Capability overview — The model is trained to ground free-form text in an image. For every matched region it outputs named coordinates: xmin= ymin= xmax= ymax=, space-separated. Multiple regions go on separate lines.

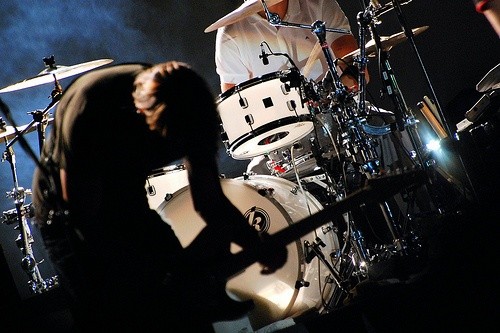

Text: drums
xmin=266 ymin=110 xmax=346 ymax=180
xmin=145 ymin=163 xmax=189 ymax=209
xmin=156 ymin=172 xmax=341 ymax=331
xmin=213 ymin=69 xmax=318 ymax=161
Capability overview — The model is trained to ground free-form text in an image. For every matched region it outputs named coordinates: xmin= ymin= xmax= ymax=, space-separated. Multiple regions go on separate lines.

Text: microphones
xmin=260 ymin=43 xmax=269 ymax=65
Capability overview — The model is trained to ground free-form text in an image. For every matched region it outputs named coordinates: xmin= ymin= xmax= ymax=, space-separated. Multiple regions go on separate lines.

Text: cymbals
xmin=204 ymin=0 xmax=284 ymax=33
xmin=0 ymin=58 xmax=114 ymax=94
xmin=337 ymin=25 xmax=428 ymax=66
xmin=0 ymin=117 xmax=54 ymax=142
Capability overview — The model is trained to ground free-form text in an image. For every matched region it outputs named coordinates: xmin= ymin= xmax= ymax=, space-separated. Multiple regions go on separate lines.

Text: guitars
xmin=62 ymin=165 xmax=427 ymax=331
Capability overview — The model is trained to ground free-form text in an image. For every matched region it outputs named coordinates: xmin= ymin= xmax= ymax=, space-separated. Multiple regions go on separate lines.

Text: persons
xmin=32 ymin=61 xmax=289 ymax=333
xmin=215 ymin=0 xmax=442 ymax=231
